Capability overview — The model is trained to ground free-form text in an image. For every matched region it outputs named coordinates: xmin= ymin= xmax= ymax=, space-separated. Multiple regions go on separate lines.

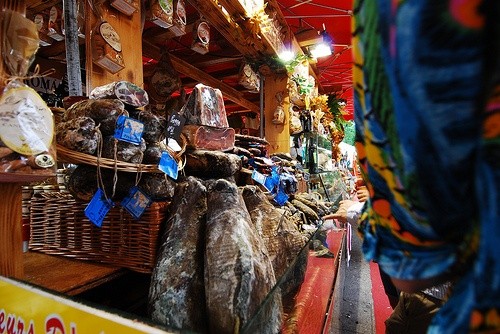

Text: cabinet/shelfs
xmin=0 ymin=0 xmax=338 ymax=312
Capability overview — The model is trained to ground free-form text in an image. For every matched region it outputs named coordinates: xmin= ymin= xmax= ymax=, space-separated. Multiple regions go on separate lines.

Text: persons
xmin=349 ymin=0 xmax=500 ymax=334
xmin=323 ymin=186 xmax=369 ymax=228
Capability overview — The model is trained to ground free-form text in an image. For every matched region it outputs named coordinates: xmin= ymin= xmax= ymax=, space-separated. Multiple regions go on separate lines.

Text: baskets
xmin=28 ymin=193 xmax=172 ymax=275
